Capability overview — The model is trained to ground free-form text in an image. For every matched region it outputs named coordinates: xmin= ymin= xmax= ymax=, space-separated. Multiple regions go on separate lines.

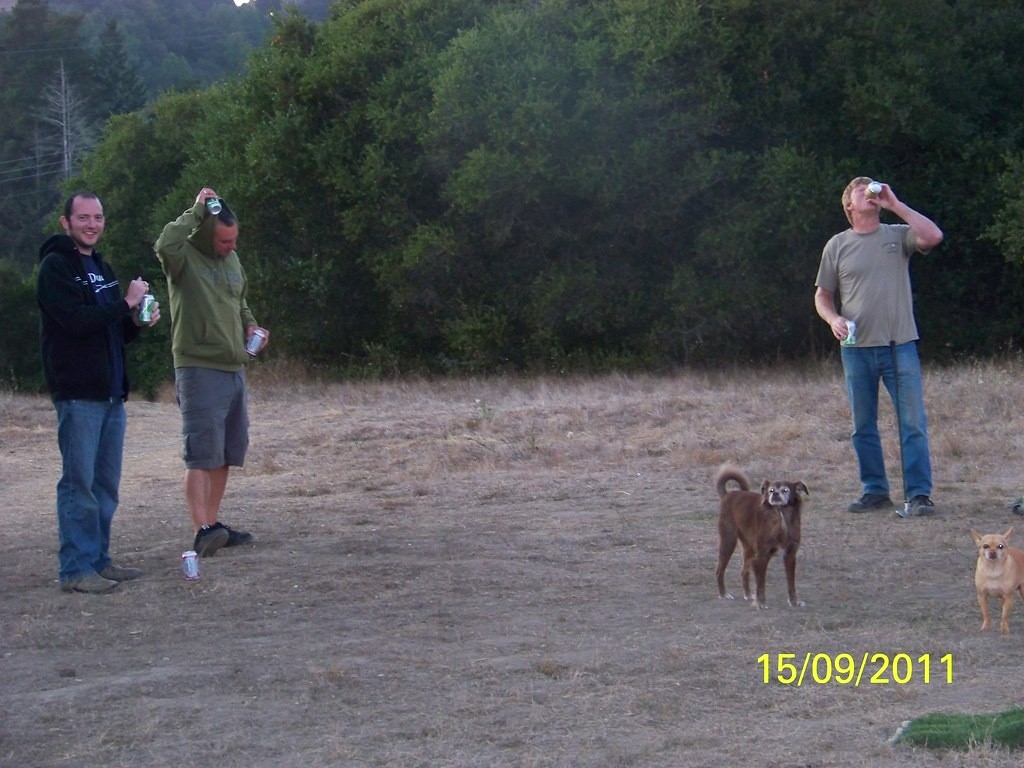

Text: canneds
xmin=137 ymin=294 xmax=155 ymax=322
xmin=181 ymin=550 xmax=200 ymax=580
xmin=205 ymin=197 xmax=222 ymax=215
xmin=864 ymin=181 xmax=882 ymax=198
xmin=841 ymin=321 xmax=856 ymax=345
xmin=244 ymin=329 xmax=265 ymax=356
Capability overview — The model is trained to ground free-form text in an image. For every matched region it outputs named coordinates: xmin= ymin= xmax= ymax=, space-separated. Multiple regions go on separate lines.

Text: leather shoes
xmin=61 ymin=572 xmax=118 ymax=594
xmin=99 ymin=562 xmax=140 ymax=581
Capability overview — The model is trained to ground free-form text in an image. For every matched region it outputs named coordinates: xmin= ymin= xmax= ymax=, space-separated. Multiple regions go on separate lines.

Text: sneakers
xmin=194 ymin=524 xmax=229 ymax=558
xmin=850 ymin=494 xmax=892 ymax=513
xmin=905 ymin=494 xmax=933 ymax=514
xmin=214 ymin=522 xmax=253 ymax=547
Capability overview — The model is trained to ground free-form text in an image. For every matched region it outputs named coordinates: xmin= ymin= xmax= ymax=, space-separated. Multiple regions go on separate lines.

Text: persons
xmin=813 ymin=177 xmax=945 ymax=518
xmin=34 ymin=191 xmax=161 ymax=594
xmin=153 ymin=188 xmax=269 ymax=558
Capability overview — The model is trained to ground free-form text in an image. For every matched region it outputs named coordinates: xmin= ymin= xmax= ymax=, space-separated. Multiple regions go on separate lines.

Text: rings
xmin=144 ymin=282 xmax=149 ymax=286
xmin=204 ymin=190 xmax=207 ymax=194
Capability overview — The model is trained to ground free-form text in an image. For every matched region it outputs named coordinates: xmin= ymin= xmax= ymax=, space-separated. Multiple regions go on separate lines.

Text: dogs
xmin=716 ymin=461 xmax=810 ymax=608
xmin=969 ymin=527 xmax=1024 ymax=635
